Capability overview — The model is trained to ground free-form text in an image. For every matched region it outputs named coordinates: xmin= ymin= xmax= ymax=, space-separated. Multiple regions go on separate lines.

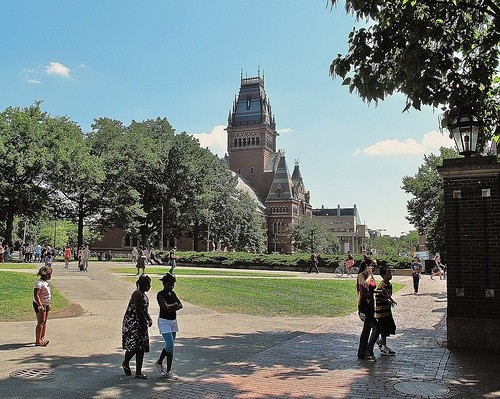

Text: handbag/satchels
xmin=79 ymin=263 xmax=85 ymax=271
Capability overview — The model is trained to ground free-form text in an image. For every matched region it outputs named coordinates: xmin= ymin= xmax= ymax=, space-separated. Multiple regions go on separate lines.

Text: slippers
xmin=135 ymin=373 xmax=150 ymax=379
xmin=122 ymin=361 xmax=132 ymax=376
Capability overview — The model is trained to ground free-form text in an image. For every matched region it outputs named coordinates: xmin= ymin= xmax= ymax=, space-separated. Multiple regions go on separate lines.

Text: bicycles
xmin=335 ymin=261 xmax=359 ymax=278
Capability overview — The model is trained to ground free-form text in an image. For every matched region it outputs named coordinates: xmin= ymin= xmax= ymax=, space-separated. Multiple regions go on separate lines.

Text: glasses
xmin=368 ymin=264 xmax=378 ymax=268
xmin=165 ymin=284 xmax=175 ymax=288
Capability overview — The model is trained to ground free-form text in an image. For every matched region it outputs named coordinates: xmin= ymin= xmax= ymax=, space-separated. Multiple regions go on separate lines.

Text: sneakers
xmin=155 ymin=361 xmax=165 ymax=376
xmin=165 ymin=370 xmax=178 ymax=380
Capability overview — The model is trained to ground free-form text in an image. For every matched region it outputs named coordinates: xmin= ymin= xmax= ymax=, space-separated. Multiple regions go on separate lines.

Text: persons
xmin=131 ymin=246 xmax=178 ymax=275
xmin=341 ymin=250 xmax=398 ymax=362
xmin=122 ymin=275 xmax=154 ymax=379
xmin=412 ymin=257 xmax=423 ymax=294
xmin=32 ymin=266 xmax=53 ymax=346
xmin=156 ymin=274 xmax=183 ymax=379
xmin=308 ymin=251 xmax=320 ymax=274
xmin=98 ymin=250 xmax=113 ymax=261
xmin=0 ymin=240 xmax=90 ymax=272
xmin=431 ymin=256 xmax=445 ymax=280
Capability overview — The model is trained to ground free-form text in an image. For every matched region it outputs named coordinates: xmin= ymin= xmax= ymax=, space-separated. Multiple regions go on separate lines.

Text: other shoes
xmin=376 ymin=337 xmax=382 ymax=350
xmin=380 ymin=346 xmax=396 ymax=355
